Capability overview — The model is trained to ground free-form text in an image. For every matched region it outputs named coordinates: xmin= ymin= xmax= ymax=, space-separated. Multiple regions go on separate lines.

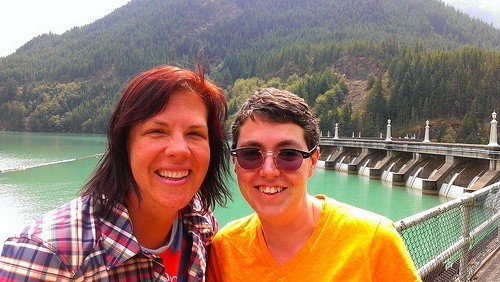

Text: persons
xmin=205 ymin=88 xmax=423 ymax=282
xmin=0 ymin=43 xmax=232 ymax=282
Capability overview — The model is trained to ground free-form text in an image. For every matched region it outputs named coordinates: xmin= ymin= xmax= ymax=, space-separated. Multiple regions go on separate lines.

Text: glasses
xmin=230 ymin=145 xmax=317 ymax=173
